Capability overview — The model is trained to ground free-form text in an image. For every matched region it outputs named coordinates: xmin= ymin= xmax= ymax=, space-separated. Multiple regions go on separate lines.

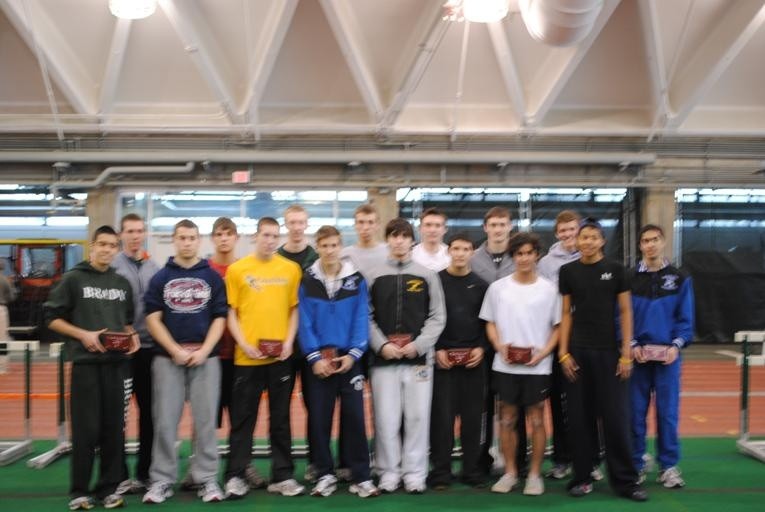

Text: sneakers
xmin=490 ymin=474 xmax=520 ymax=494
xmin=569 ymin=483 xmax=593 ymax=497
xmin=116 ymin=480 xmax=134 ymax=496
xmin=244 ymin=465 xmax=265 ymax=490
xmin=266 ymin=479 xmax=305 ymax=498
xmin=625 ymin=489 xmax=648 ymax=502
xmin=309 ymin=475 xmax=338 ymax=498
xmin=469 ymin=480 xmax=489 ymax=490
xmin=522 ymin=475 xmax=545 ymax=497
xmin=591 ymin=469 xmax=604 ymax=482
xmin=655 ymin=467 xmax=685 ymax=488
xmin=196 ymin=481 xmax=224 ymax=504
xmin=222 ymin=477 xmax=250 ymax=498
xmin=546 ymin=465 xmax=573 ymax=480
xmin=433 ymin=477 xmax=452 ymax=492
xmin=378 ymin=479 xmax=399 ymax=493
xmin=101 ymin=494 xmax=126 ymax=509
xmin=403 ymin=477 xmax=427 ymax=494
xmin=141 ymin=481 xmax=175 ymax=504
xmin=68 ymin=496 xmax=95 ymax=510
xmin=349 ymin=480 xmax=380 ymax=498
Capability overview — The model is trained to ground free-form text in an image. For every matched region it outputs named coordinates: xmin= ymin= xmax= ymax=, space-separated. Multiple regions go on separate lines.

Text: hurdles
xmin=735 ymin=331 xmax=764 ymax=459
xmin=0 ymin=339 xmax=40 ymax=466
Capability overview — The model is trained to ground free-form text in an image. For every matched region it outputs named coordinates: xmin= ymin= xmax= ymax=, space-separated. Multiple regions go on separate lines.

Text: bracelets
xmin=557 ymin=351 xmax=572 ymax=367
xmin=617 ymin=355 xmax=635 ymax=370
xmin=128 ymin=328 xmax=137 ymax=336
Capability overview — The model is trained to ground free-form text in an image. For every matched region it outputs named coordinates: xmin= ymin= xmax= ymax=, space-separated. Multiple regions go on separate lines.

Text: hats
xmin=579 ymin=216 xmax=604 ymax=235
xmin=444 ymin=229 xmax=489 ymax=249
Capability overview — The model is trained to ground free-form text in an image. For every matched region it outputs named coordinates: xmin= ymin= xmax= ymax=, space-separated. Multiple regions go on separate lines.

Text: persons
xmin=108 ymin=214 xmax=163 ymax=497
xmin=410 ymin=205 xmax=453 ymax=271
xmin=478 ymin=232 xmax=563 ymax=495
xmin=0 ymin=260 xmax=18 ymax=355
xmin=224 ymin=217 xmax=306 ymax=499
xmin=178 ymin=217 xmax=266 ymax=489
xmin=297 ymin=225 xmax=381 ymax=498
xmin=617 ymin=224 xmax=692 ymax=487
xmin=42 ymin=225 xmax=141 ymax=510
xmin=470 ymin=207 xmax=531 ymax=479
xmin=336 ymin=205 xmax=390 ymax=482
xmin=425 ymin=235 xmax=495 ymax=491
xmin=277 ymin=206 xmax=319 ymax=482
xmin=536 ymin=209 xmax=606 ymax=481
xmin=558 ymin=217 xmax=649 ymax=501
xmin=366 ymin=218 xmax=447 ymax=493
xmin=143 ymin=220 xmax=228 ymax=503
